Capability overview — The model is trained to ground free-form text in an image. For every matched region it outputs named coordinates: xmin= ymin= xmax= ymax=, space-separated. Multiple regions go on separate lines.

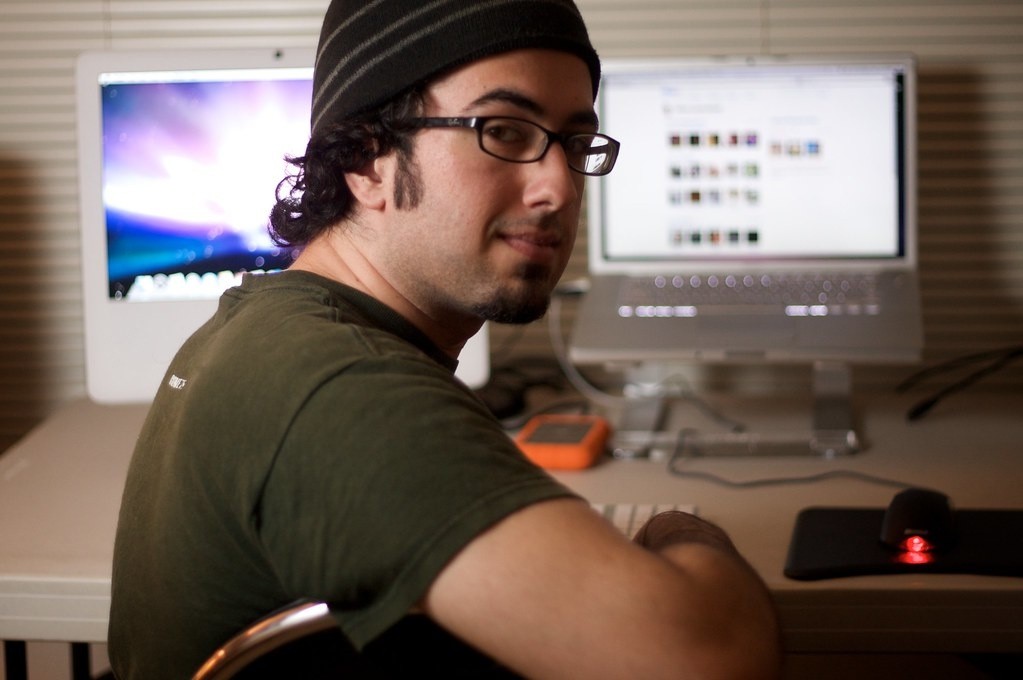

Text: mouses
xmin=881 ymin=485 xmax=952 ymax=559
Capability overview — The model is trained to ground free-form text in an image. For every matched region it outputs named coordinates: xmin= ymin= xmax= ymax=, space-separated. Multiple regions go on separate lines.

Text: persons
xmin=108 ymin=1 xmax=778 ymax=680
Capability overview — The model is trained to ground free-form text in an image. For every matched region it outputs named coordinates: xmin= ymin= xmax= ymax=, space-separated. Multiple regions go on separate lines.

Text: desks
xmin=0 ymin=398 xmax=1023 ymax=680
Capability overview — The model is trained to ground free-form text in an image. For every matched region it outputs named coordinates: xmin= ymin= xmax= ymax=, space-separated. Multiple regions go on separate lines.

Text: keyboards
xmin=587 ymin=498 xmax=698 ymax=545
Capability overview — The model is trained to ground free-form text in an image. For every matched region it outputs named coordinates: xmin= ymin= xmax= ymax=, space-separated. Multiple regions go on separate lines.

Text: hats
xmin=310 ymin=0 xmax=601 ymax=137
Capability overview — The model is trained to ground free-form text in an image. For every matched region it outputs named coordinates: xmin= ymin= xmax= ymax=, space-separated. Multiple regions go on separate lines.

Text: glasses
xmin=380 ymin=115 xmax=621 ymax=176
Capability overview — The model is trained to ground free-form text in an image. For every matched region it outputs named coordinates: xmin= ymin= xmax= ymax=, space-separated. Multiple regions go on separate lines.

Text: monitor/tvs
xmin=75 ymin=47 xmax=487 ymax=402
xmin=570 ymin=56 xmax=916 ymax=450
